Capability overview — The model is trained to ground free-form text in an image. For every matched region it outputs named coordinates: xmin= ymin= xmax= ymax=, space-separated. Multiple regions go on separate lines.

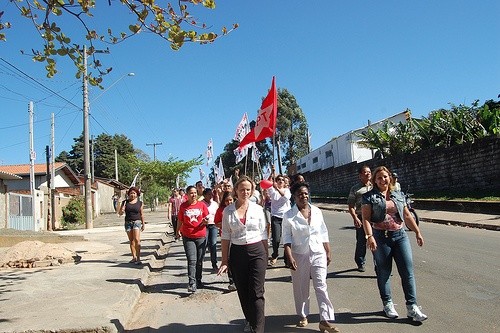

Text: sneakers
xmin=383 ymin=302 xmax=400 ymax=318
xmin=408 ymin=305 xmax=428 ymax=321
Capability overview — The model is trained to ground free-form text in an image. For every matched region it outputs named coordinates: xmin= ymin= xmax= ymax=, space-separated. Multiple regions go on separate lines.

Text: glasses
xmin=224 ymin=184 xmax=231 ymax=187
xmin=277 ymin=178 xmax=285 ymax=181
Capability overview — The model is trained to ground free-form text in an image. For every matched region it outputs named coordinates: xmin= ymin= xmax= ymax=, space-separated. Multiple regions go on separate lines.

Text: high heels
xmin=319 ymin=322 xmax=339 ymax=332
xmin=298 ymin=318 xmax=309 ymax=327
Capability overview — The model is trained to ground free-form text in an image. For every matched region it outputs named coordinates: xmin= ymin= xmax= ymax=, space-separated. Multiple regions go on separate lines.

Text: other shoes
xmin=243 ymin=321 xmax=251 ymax=331
xmin=129 ymin=258 xmax=136 ymax=263
xmin=188 ymin=284 xmax=198 ymax=295
xmin=197 ymin=280 xmax=204 ymax=286
xmin=136 ymin=260 xmax=143 ymax=265
xmin=227 ymin=282 xmax=237 ymax=291
xmin=357 ymin=264 xmax=365 ymax=272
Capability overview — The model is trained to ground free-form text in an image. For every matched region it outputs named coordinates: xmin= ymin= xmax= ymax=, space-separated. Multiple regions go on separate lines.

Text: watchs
xmin=365 ymin=234 xmax=373 ymax=239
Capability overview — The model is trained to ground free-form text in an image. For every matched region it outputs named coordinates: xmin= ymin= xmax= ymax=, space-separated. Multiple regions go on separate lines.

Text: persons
xmin=347 ymin=166 xmax=379 ymax=272
xmin=392 ymin=172 xmax=402 ymax=192
xmin=166 ymin=178 xmax=238 ymax=292
xmin=236 ymin=164 xmax=311 ymax=268
xmin=111 ymin=193 xmax=119 ymax=211
xmin=116 ymin=187 xmax=145 ymax=265
xmin=360 ymin=166 xmax=429 ymax=322
xmin=215 ymin=177 xmax=269 ymax=333
xmin=281 ymin=182 xmax=340 ymax=333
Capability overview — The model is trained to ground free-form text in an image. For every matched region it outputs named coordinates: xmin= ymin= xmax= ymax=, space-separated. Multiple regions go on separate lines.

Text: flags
xmin=233 ymin=144 xmax=247 ymax=164
xmin=250 ymin=145 xmax=263 ymax=163
xmin=232 ymin=112 xmax=254 ymax=149
xmin=239 ymin=78 xmax=277 ymax=150
xmin=199 ymin=159 xmax=225 ymax=187
xmin=206 ymin=140 xmax=213 ymax=158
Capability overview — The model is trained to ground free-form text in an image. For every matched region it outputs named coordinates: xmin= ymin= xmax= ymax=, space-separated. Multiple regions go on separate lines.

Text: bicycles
xmin=404 ymin=193 xmax=419 ymax=230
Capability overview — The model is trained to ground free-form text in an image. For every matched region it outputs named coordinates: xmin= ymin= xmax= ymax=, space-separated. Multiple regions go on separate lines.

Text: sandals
xmin=270 ymin=256 xmax=278 ymax=265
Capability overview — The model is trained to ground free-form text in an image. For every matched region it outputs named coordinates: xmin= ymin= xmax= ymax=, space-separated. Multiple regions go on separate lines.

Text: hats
xmin=392 ymin=173 xmax=399 ymax=177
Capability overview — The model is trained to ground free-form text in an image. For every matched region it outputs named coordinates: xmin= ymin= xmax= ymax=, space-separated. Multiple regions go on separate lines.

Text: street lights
xmin=83 ymin=71 xmax=136 ymax=229
